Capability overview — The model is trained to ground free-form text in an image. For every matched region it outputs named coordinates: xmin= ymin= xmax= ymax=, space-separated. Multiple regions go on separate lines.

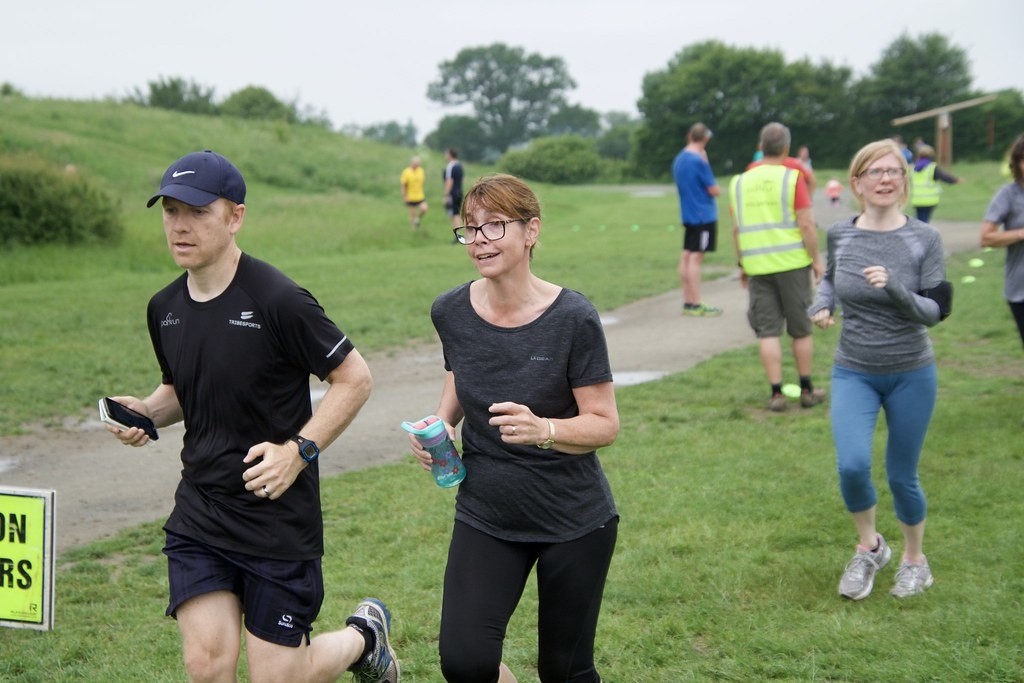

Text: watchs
xmin=537 ymin=417 xmax=556 ymax=449
xmin=290 ymin=435 xmax=319 ymax=462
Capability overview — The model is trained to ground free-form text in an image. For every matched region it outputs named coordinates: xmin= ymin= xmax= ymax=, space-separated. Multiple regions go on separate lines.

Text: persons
xmin=894 ymin=136 xmax=924 ymax=163
xmin=107 ymin=152 xmax=400 ymax=683
xmin=744 ymin=138 xmax=816 ymax=198
xmin=725 ymin=120 xmax=826 ymax=411
xmin=409 ymin=173 xmax=619 ymax=683
xmin=978 ymin=133 xmax=1024 ymax=347
xmin=806 ymin=139 xmax=952 ymax=601
xmin=906 ymin=144 xmax=960 ymax=223
xmin=669 ymin=123 xmax=723 ymax=316
xmin=826 ymin=178 xmax=843 ymax=204
xmin=442 ymin=147 xmax=464 ymax=245
xmin=401 ymin=156 xmax=428 ymax=226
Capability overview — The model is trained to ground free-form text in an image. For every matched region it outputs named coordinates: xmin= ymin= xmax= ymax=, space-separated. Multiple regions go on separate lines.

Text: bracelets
xmin=738 ymin=262 xmax=743 ymax=267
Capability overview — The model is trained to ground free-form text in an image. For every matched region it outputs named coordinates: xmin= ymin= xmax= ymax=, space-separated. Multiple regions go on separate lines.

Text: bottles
xmin=402 ymin=415 xmax=467 ymax=488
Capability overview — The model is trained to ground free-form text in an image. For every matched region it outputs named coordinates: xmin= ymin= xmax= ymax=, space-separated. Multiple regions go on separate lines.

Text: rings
xmin=512 ymin=426 xmax=515 ymax=434
xmin=262 ymin=486 xmax=270 ymax=496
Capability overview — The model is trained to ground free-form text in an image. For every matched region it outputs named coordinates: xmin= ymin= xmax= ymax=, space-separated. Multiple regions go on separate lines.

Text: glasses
xmin=861 ymin=168 xmax=904 ymax=180
xmin=452 ymin=218 xmax=522 ymax=245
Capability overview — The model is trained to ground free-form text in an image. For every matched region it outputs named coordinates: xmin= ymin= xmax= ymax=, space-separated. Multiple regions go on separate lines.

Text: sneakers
xmin=682 ymin=302 xmax=721 ymax=318
xmin=837 ymin=534 xmax=891 ymax=600
xmin=799 ymin=389 xmax=828 ymax=407
xmin=345 ymin=599 xmax=401 ymax=683
xmin=888 ymin=554 xmax=934 ymax=599
xmin=769 ymin=396 xmax=786 ymax=411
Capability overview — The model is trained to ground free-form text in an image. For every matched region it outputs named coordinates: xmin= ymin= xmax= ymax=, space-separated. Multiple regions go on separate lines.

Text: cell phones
xmin=103 ymin=395 xmax=159 ymax=442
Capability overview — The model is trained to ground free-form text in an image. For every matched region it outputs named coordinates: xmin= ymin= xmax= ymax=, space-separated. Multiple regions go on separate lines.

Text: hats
xmin=147 ymin=149 xmax=246 ymax=206
